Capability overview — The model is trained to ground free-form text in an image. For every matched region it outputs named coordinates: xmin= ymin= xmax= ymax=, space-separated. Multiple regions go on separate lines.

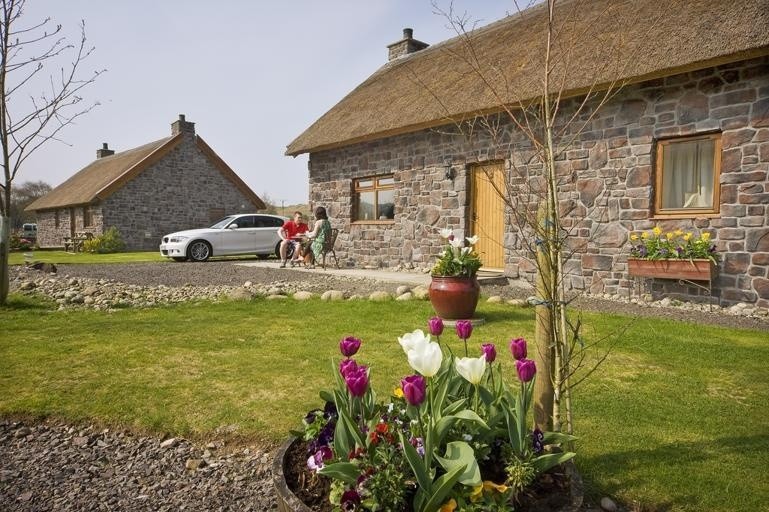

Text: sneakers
xmin=279 ymin=259 xmax=316 ymax=269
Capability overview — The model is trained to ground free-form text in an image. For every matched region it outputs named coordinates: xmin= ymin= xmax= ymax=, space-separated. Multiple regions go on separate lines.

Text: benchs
xmin=64 ymin=231 xmax=94 ymax=253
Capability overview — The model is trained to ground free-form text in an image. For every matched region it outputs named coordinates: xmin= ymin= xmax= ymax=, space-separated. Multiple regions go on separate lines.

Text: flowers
xmin=289 ymin=314 xmax=580 ymax=511
xmin=428 ymin=228 xmax=484 ymax=280
xmin=624 ymin=224 xmax=723 ymax=267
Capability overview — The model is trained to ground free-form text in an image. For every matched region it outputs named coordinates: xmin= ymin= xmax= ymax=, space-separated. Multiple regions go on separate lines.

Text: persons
xmin=293 ymin=207 xmax=332 ymax=269
xmin=277 ymin=211 xmax=309 ymax=268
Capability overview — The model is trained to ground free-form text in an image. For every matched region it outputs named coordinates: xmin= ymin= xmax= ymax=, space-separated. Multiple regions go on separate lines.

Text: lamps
xmin=442 ymin=160 xmax=453 ymax=181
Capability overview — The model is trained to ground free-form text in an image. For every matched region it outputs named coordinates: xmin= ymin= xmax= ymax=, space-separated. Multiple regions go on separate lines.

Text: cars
xmin=159 ymin=214 xmax=295 ymax=261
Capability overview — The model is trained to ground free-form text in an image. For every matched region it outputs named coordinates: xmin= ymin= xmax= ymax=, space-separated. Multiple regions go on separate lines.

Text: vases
xmin=270 ymin=429 xmax=584 ymax=511
xmin=429 ymin=274 xmax=480 ymax=320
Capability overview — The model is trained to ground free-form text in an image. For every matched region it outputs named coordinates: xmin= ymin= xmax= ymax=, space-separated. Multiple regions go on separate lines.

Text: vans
xmin=22 ymin=224 xmax=37 ymax=237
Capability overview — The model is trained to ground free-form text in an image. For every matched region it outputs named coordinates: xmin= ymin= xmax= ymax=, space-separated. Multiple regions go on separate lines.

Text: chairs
xmin=285 ymin=228 xmax=340 ymax=271
xmin=240 ymin=219 xmax=273 ymax=229
